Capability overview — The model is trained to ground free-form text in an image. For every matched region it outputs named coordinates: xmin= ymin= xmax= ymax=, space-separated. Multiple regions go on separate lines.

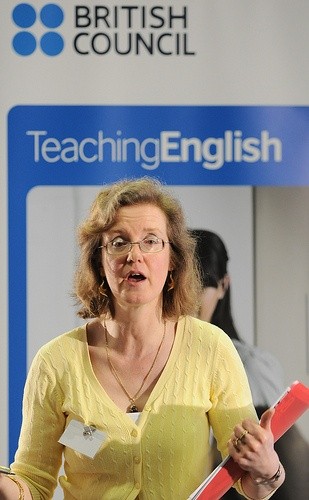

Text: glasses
xmin=97 ymin=236 xmax=170 ymax=254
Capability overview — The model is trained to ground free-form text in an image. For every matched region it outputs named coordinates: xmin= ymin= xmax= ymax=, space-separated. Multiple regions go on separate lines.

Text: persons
xmin=0 ymin=174 xmax=287 ymax=500
xmin=187 ymin=228 xmax=309 ymax=500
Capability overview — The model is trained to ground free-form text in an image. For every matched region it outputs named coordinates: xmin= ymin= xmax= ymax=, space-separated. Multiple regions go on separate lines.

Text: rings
xmin=233 ymin=438 xmax=238 ymax=447
xmin=239 ymin=430 xmax=248 ymax=439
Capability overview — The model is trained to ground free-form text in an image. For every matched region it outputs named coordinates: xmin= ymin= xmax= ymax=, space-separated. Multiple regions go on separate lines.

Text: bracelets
xmin=251 ymin=463 xmax=282 ymax=484
xmin=7 ymin=476 xmax=24 ymax=500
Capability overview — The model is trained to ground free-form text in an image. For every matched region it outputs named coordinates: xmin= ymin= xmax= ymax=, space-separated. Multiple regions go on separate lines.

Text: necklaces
xmin=104 ymin=315 xmax=166 ymax=412
